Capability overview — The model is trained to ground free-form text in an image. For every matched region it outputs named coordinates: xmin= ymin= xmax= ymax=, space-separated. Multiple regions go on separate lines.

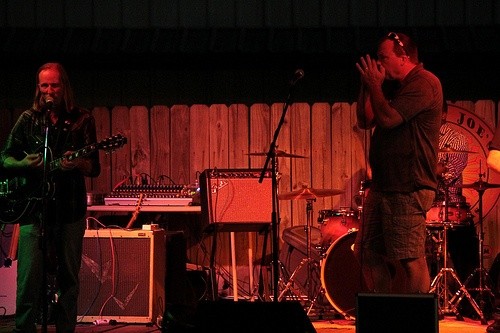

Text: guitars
xmin=0 ymin=134 xmax=127 ymax=223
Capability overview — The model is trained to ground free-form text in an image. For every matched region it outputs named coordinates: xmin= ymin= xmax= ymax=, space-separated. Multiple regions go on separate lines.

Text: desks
xmin=86 ymin=204 xmax=201 ymax=228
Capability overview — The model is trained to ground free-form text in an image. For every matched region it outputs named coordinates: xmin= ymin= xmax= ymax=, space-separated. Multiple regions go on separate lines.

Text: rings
xmin=364 ymin=66 xmax=368 ymax=70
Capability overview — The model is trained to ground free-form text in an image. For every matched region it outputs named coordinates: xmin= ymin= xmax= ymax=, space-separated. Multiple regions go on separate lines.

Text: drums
xmin=318 ymin=207 xmax=364 ymax=256
xmin=321 ymin=228 xmax=367 ymax=320
xmin=424 ymin=201 xmax=471 ymax=229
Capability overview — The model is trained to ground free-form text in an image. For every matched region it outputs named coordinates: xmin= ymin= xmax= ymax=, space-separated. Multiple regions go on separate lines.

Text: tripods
xmin=429 ymin=152 xmax=494 ymax=325
xmin=277 ymin=198 xmax=322 ymax=302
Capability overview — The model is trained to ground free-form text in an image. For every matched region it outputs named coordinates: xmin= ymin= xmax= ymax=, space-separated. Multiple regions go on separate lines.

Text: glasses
xmin=386 ymin=32 xmax=408 ymax=56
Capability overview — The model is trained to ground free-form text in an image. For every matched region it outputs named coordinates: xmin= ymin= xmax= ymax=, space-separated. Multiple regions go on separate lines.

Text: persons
xmin=0 ymin=62 xmax=101 ymax=333
xmin=356 ymin=32 xmax=443 ymax=294
xmin=436 ymin=99 xmax=468 ymax=207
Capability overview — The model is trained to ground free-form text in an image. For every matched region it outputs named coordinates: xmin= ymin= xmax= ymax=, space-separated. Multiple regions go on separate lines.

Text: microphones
xmin=291 ymin=70 xmax=304 ymax=84
xmin=45 ymin=97 xmax=55 ymax=112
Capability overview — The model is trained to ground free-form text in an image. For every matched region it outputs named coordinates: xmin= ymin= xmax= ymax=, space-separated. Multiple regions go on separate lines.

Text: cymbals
xmin=242 ymin=149 xmax=309 ymax=159
xmin=279 ymin=187 xmax=343 ymax=200
xmin=449 ymin=180 xmax=500 ymax=191
xmin=438 ymin=147 xmax=480 ymax=154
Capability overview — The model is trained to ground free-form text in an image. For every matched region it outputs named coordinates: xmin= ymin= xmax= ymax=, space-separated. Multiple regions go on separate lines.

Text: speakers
xmin=76 ymin=228 xmax=168 ymax=324
xmin=160 ymin=300 xmax=318 ymax=333
xmin=200 ymin=168 xmax=274 ymax=234
xmin=354 ymin=294 xmax=439 ymax=333
